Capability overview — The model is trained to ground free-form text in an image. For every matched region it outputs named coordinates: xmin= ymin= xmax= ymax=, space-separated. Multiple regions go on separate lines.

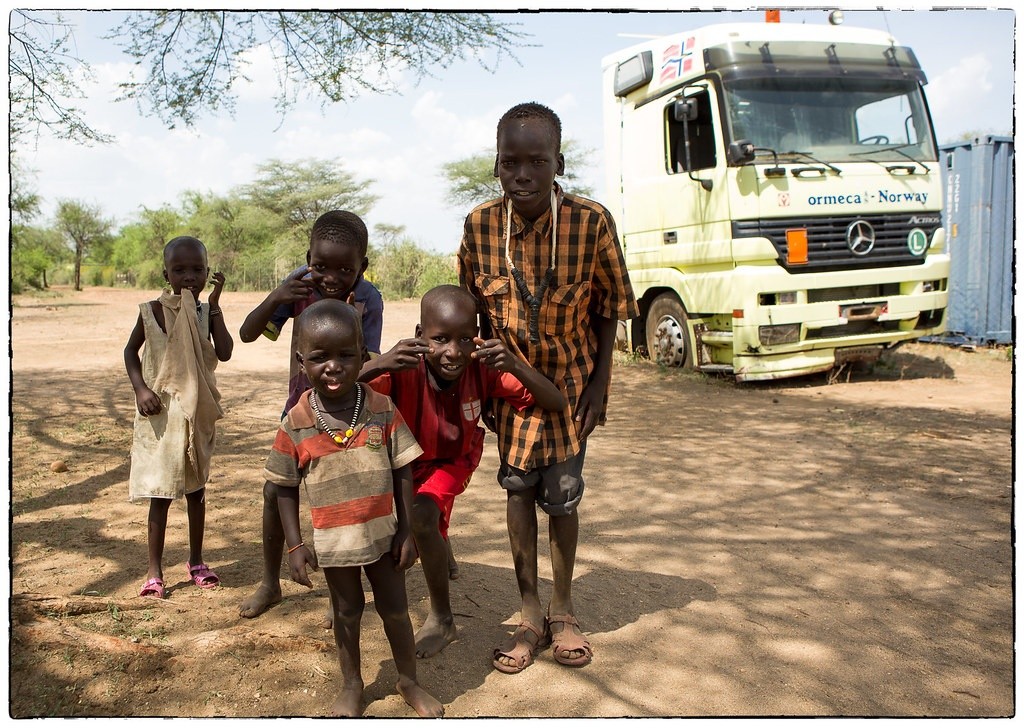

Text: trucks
xmin=602 ymin=11 xmax=951 ymax=384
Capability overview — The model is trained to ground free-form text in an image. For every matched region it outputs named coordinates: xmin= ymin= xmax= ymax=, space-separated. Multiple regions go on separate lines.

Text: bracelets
xmin=287 ymin=542 xmax=307 ymax=554
xmin=210 ymin=309 xmax=222 ymax=316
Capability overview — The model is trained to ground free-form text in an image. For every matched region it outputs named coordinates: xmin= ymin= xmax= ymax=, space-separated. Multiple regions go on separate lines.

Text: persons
xmin=238 ymin=212 xmax=568 ymax=719
xmin=124 ymin=236 xmax=234 ymax=598
xmin=459 ymin=102 xmax=640 ymax=675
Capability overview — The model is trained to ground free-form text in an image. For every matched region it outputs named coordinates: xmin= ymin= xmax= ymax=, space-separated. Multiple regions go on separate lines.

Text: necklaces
xmin=194 ymin=300 xmax=202 ymax=313
xmin=311 ymin=382 xmax=362 ymax=443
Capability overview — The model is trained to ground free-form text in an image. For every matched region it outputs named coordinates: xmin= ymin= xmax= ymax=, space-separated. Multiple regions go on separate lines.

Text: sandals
xmin=185 ymin=557 xmax=221 ymax=589
xmin=547 ymin=599 xmax=591 ymax=666
xmin=138 ymin=578 xmax=165 ymax=599
xmin=492 ymin=618 xmax=549 ymax=673
